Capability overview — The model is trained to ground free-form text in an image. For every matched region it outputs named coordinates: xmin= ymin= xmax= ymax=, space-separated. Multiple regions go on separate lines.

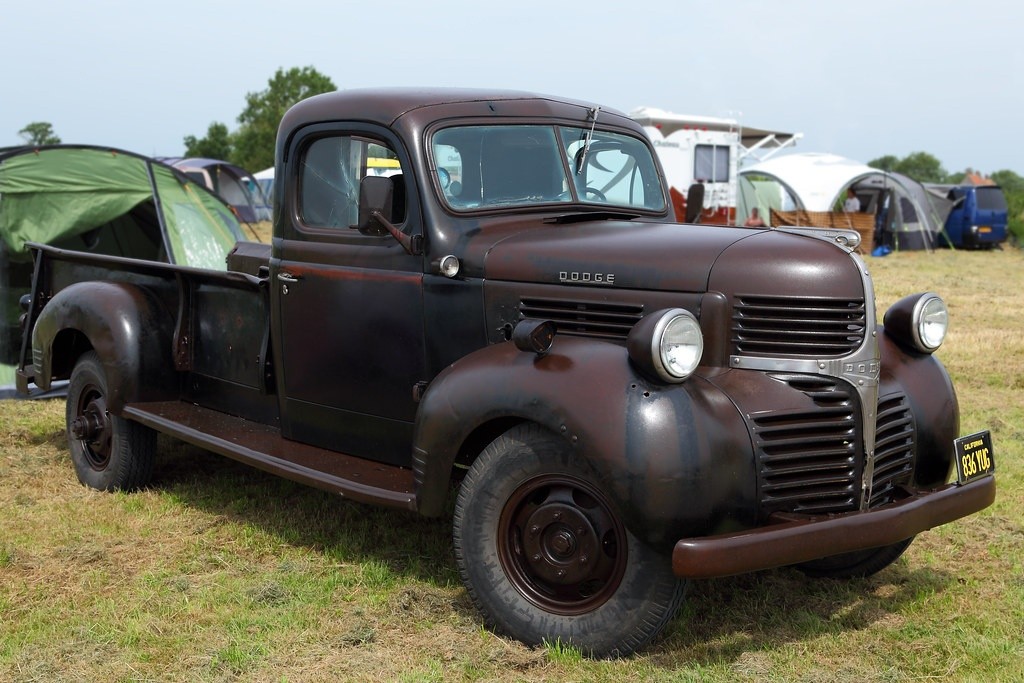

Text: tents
xmin=150 ymin=156 xmax=274 ymax=226
xmin=734 ymin=152 xmax=957 ymax=253
xmin=240 ymin=155 xmax=404 ymax=219
xmin=0 ymin=145 xmax=266 ymax=397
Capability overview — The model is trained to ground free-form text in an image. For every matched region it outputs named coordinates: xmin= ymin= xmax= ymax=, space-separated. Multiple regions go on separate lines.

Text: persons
xmin=844 ymin=188 xmax=860 ymax=213
xmin=747 ymin=206 xmax=765 ymax=227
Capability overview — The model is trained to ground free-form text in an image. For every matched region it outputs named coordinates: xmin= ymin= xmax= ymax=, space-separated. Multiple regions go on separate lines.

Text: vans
xmin=878 ymin=183 xmax=1008 ymax=250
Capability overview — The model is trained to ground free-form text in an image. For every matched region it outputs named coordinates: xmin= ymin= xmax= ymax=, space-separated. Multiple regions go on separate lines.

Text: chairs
xmin=313 ymin=173 xmax=520 ymax=229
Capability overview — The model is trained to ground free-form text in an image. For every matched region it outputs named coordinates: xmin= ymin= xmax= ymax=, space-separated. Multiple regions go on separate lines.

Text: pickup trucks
xmin=17 ymin=82 xmax=997 ymax=662
